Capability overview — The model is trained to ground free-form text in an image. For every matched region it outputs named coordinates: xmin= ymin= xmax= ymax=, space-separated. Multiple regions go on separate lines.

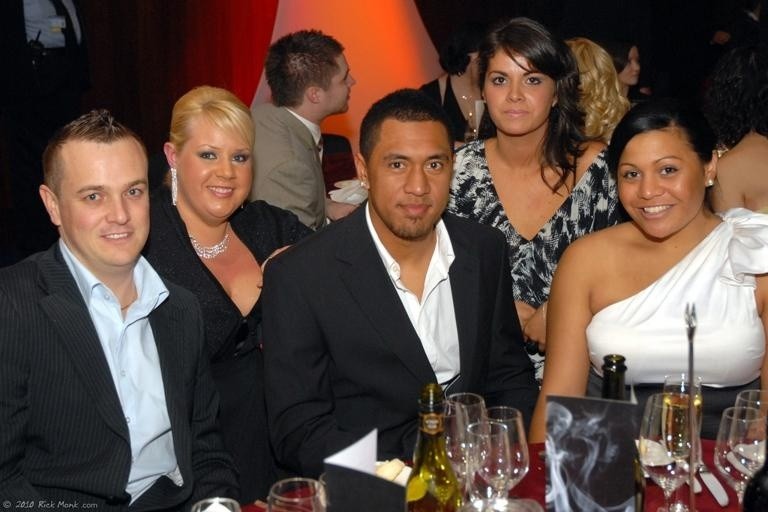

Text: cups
xmin=263 ymin=472 xmax=326 ymax=512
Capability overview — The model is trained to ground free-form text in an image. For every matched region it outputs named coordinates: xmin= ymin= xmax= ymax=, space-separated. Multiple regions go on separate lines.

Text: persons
xmin=446 ymin=17 xmax=624 ymax=391
xmin=140 ymin=83 xmax=319 ymax=507
xmin=1 ymin=109 xmax=240 ymax=511
xmin=0 ymin=1 xmax=768 ymax=234
xmin=527 ymin=92 xmax=768 ymax=445
xmin=258 ymin=88 xmax=541 ymax=481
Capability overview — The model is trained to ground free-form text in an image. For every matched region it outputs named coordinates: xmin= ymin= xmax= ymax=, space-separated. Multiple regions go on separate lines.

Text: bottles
xmin=402 ymin=383 xmax=458 ymax=512
xmin=598 ymin=352 xmax=629 ymax=402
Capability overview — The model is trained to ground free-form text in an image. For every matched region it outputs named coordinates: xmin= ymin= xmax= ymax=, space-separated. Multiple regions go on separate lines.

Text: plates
xmin=376 ymin=458 xmax=414 ymax=486
xmin=329 ymin=181 xmax=367 ymax=206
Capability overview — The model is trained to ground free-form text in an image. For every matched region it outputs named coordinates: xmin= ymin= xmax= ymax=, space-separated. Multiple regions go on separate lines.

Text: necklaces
xmin=185 ymin=222 xmax=231 ymax=261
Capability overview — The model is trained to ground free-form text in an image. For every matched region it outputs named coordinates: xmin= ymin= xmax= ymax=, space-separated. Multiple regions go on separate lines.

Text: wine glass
xmin=442 ymin=393 xmax=531 ymax=511
xmin=640 ymin=372 xmax=764 ymax=512
